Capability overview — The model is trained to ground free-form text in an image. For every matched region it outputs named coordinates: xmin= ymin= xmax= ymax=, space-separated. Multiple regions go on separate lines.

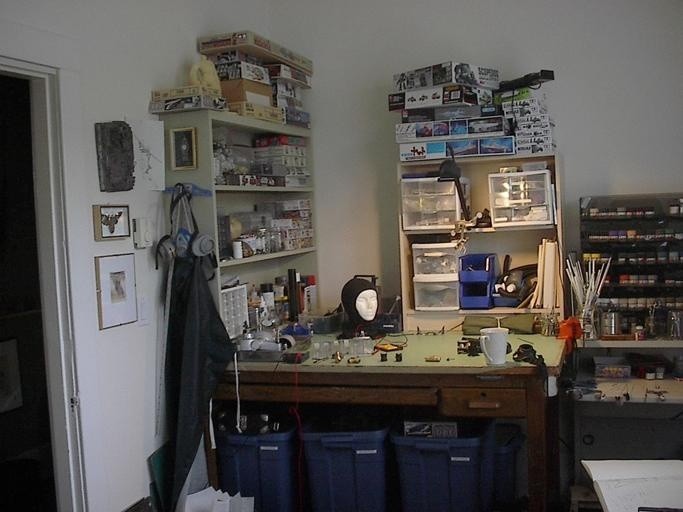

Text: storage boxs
xmin=297 ymin=305 xmax=347 ymax=334
xmin=388 ymin=58 xmax=557 ymax=163
xmin=197 ymin=30 xmax=312 ymax=128
xmin=369 ymin=296 xmax=402 ymax=333
xmin=213 ymin=409 xmax=295 ymax=512
xmin=391 ymin=418 xmax=483 ymax=511
xmin=221 ymin=283 xmax=250 ymax=339
xmin=401 ymin=178 xmax=471 ymax=229
xmin=487 ymin=169 xmax=554 ymax=228
xmin=295 ymin=414 xmax=391 ymax=512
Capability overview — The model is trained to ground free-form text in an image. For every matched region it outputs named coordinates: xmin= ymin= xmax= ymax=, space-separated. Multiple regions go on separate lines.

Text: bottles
xmin=634 ymin=325 xmax=644 ymax=341
xmin=269 ymin=226 xmax=282 ymax=252
xmin=254 ymin=228 xmax=268 ymax=255
xmin=656 ymin=367 xmax=665 ymax=379
xmin=645 ymin=368 xmax=656 ymax=379
xmin=581 ymin=204 xmax=682 ymax=312
xmin=310 ymin=335 xmax=370 ymax=360
xmin=639 ymin=367 xmax=645 ymax=377
xmin=247 ymin=266 xmax=317 ymax=332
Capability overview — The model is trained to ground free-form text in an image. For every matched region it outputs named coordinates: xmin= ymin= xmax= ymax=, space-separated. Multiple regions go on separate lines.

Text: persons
xmin=338 ymin=277 xmax=384 ymax=340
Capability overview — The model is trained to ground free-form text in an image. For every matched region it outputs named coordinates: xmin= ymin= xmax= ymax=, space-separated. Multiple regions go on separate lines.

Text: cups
xmin=478 ymin=327 xmax=509 ymax=365
xmin=600 ymin=311 xmax=619 ymax=335
xmin=231 ymin=240 xmax=242 ymax=258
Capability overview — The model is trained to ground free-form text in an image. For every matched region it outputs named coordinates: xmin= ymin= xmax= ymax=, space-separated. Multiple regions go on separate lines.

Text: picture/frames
xmin=94 ymin=252 xmax=138 ymax=331
xmin=0 ymin=335 xmax=24 ymax=415
xmin=93 ymin=203 xmax=132 ymax=241
xmin=169 ymin=125 xmax=197 ymax=172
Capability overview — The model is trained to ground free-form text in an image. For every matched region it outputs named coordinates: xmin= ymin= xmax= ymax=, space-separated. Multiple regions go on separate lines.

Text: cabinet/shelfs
xmin=158 ymin=109 xmax=321 ymax=342
xmin=395 ymin=151 xmax=566 ymax=335
xmin=411 ymin=240 xmax=460 ymax=312
xmin=570 ymin=191 xmax=683 ymax=511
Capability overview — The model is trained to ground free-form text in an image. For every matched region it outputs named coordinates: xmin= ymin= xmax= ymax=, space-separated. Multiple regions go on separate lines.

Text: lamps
xmin=438 ymin=141 xmax=469 ymax=230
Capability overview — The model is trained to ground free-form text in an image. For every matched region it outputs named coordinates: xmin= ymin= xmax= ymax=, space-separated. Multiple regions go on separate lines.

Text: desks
xmin=203 ymin=332 xmax=566 ymax=512
